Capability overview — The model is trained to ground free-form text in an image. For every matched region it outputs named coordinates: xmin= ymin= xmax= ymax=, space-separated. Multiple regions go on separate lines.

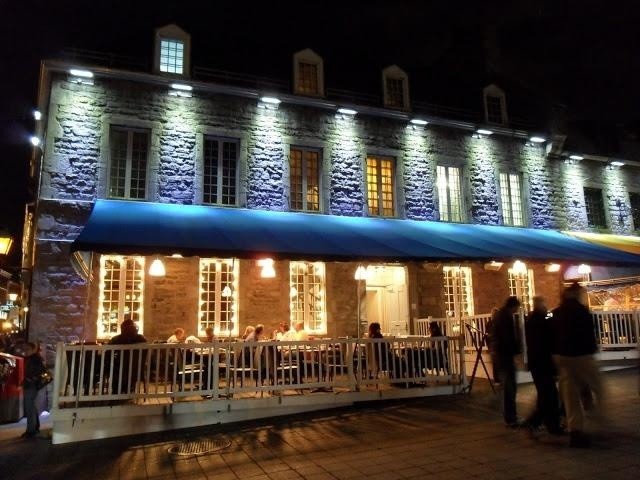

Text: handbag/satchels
xmin=32 ymin=369 xmax=53 ymax=390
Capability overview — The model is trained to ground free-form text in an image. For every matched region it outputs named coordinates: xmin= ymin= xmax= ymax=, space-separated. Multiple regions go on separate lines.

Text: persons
xmin=166 ymin=320 xmax=308 ymax=378
xmin=414 ymin=322 xmax=446 ymax=380
xmin=23 ymin=342 xmax=43 ymax=437
xmin=367 ymin=322 xmax=404 ymax=384
xmin=492 ymin=296 xmax=525 ymax=427
xmin=521 ymin=296 xmax=567 ymax=437
xmin=558 ymin=287 xmax=601 ymax=417
xmin=554 ymin=282 xmax=598 ymax=447
xmin=108 ymin=319 xmax=146 ymax=345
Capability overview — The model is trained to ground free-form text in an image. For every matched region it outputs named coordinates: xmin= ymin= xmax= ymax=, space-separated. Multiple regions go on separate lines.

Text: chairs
xmin=473 ymin=311 xmax=634 ymax=345
xmin=65 ymin=333 xmax=450 ymax=397
